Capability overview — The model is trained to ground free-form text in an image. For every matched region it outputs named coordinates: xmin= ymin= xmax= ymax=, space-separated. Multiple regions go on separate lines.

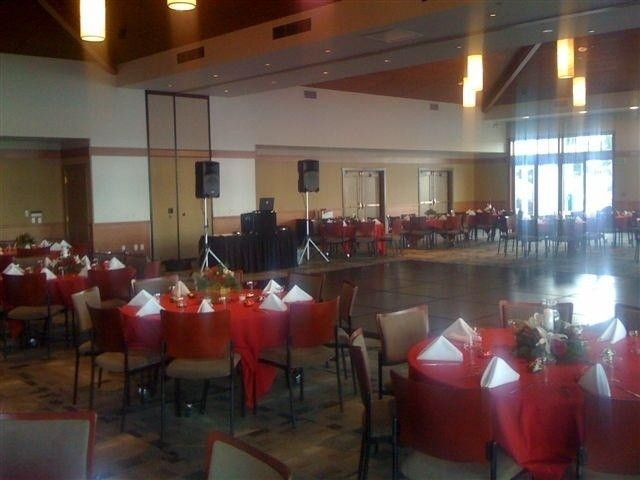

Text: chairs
xmin=499 ymin=300 xmax=573 ymax=330
xmin=484 ymin=442 xmax=589 ymax=480
xmin=615 ymin=303 xmax=640 ymax=332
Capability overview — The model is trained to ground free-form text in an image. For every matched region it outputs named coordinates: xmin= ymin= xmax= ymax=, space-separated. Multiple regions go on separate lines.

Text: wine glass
xmin=629 ymin=330 xmax=640 ymax=352
xmin=463 ymin=343 xmax=479 ymax=368
xmin=508 ymin=319 xmax=519 ymax=336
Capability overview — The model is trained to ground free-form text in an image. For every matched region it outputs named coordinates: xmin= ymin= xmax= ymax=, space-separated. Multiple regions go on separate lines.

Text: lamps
xmin=557 ymin=38 xmax=574 ymax=79
xmin=572 ymin=77 xmax=586 ymax=106
xmin=467 ymin=55 xmax=483 ymax=91
xmin=462 ymin=77 xmax=476 ymax=108
xmin=80 ymin=0 xmax=105 ymax=42
xmin=167 ymin=0 xmax=197 ymax=11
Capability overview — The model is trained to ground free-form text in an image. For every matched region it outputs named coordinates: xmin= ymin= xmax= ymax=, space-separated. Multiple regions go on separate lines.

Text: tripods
xmin=199 ymin=203 xmax=229 ymax=272
xmin=297 ymin=196 xmax=330 ymax=264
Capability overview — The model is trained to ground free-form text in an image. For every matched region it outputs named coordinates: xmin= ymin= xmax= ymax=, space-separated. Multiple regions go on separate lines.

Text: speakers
xmin=297 ymin=159 xmax=320 ymax=192
xmin=195 ymin=161 xmax=220 ymax=198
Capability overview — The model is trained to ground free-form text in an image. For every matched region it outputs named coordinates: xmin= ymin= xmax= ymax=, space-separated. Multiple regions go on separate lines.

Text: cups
xmin=121 ymin=244 xmax=145 ymax=255
xmin=239 ymin=292 xmax=247 ymax=303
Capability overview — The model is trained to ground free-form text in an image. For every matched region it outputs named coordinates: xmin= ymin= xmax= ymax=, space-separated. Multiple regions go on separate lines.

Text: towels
xmin=577 ymin=363 xmax=612 ymax=397
xmin=599 ymin=319 xmax=627 ymax=344
xmin=50 ymin=242 xmax=62 ymax=252
xmin=24 ymin=243 xmax=31 ymax=249
xmin=38 ymin=239 xmax=52 ymax=248
xmin=60 ymin=240 xmax=71 ymax=249
